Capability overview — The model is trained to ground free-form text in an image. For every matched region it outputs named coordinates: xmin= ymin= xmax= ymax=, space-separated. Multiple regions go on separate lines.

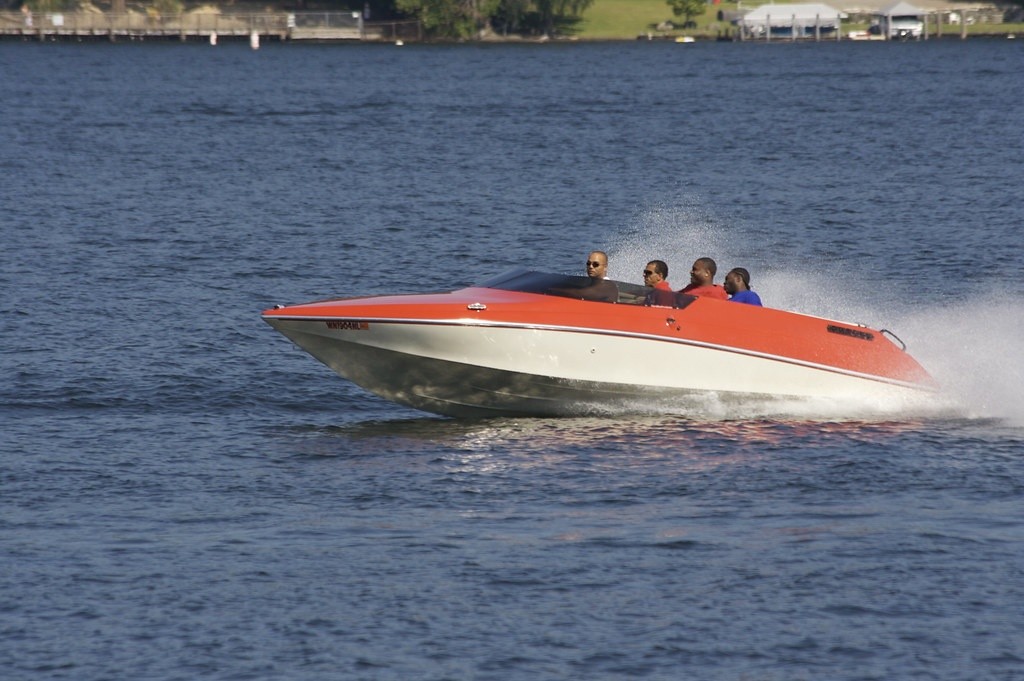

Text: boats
xmin=260 ymin=265 xmax=965 ymax=425
xmin=674 ymin=35 xmax=696 ymax=44
xmin=848 ymin=30 xmax=887 ymax=43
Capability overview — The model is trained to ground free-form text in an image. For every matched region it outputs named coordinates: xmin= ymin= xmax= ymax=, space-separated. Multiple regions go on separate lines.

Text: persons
xmin=546 ymin=250 xmax=618 ymax=302
xmin=723 ymin=267 xmax=763 ymax=307
xmin=641 ymin=260 xmax=677 ymax=309
xmin=20 ymin=3 xmax=31 ymax=29
xmin=680 ymin=257 xmax=728 ymax=301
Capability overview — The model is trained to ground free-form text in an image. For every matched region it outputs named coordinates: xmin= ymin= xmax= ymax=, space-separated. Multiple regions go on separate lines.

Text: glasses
xmin=644 ymin=270 xmax=661 ymax=276
xmin=586 ymin=261 xmax=606 ymax=268
